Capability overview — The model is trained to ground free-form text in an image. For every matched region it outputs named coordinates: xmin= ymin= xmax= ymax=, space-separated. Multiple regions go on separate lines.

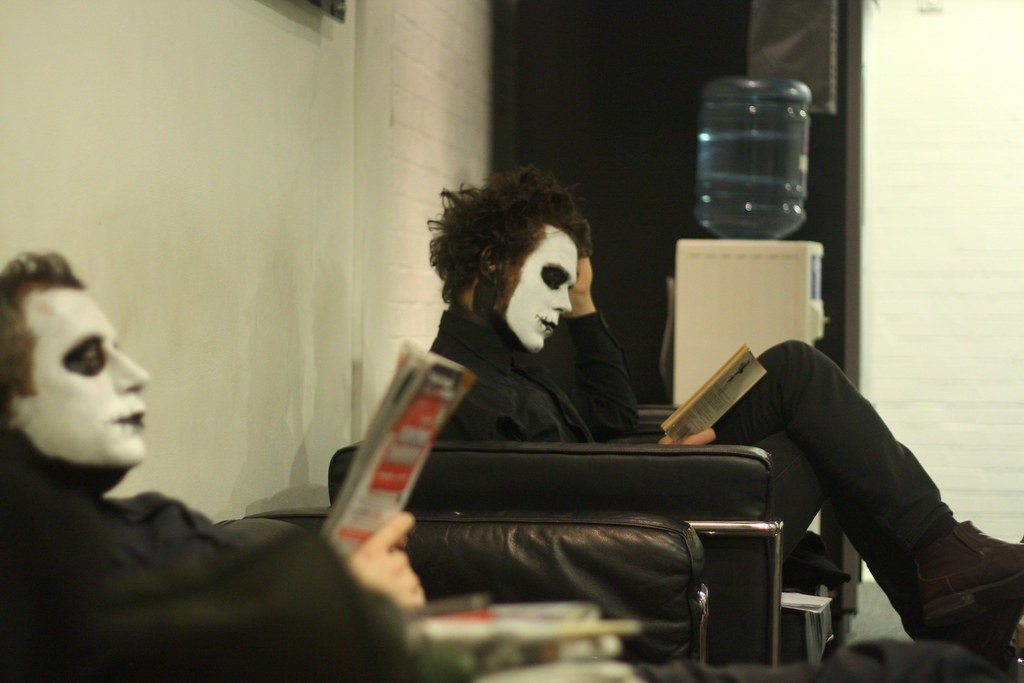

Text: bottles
xmin=695 ymin=75 xmax=812 ymax=241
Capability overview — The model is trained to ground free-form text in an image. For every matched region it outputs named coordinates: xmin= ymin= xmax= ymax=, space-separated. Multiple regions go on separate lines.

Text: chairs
xmin=217 ymin=402 xmax=866 ymax=683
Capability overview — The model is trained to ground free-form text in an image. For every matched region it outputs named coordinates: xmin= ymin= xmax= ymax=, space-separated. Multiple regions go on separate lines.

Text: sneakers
xmin=923 ymin=597 xmax=1024 ymax=667
xmin=916 ymin=522 xmax=1024 ymax=627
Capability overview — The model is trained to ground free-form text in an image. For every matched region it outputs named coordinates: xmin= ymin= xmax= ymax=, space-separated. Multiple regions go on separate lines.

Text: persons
xmin=425 ymin=170 xmax=1023 ymax=640
xmin=0 ymin=255 xmax=475 ymax=683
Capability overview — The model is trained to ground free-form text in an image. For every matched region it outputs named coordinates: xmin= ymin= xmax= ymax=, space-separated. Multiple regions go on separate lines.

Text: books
xmin=660 ymin=343 xmax=767 ymax=442
xmin=321 ymin=337 xmax=474 ymax=556
